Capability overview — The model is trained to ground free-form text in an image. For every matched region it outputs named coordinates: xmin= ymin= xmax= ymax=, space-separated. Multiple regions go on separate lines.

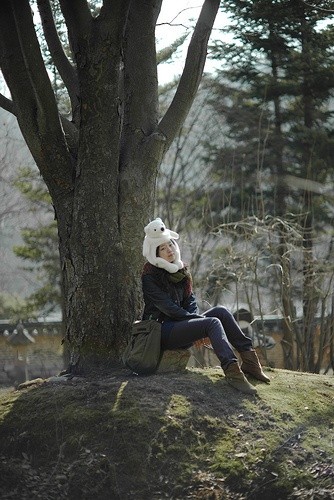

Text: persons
xmin=143 ymin=218 xmax=271 ymax=393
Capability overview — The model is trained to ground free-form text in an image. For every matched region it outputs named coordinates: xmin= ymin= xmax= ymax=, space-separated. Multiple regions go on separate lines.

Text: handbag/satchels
xmin=121 ymin=319 xmax=162 ymax=375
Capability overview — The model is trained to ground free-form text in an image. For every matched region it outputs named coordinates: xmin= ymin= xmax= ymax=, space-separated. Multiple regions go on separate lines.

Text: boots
xmin=239 ymin=351 xmax=271 ymax=383
xmin=224 ymin=362 xmax=257 ymax=395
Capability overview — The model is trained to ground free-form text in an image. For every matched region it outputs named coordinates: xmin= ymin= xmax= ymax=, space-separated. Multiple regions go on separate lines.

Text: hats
xmin=142 ymin=217 xmax=184 ymax=273
